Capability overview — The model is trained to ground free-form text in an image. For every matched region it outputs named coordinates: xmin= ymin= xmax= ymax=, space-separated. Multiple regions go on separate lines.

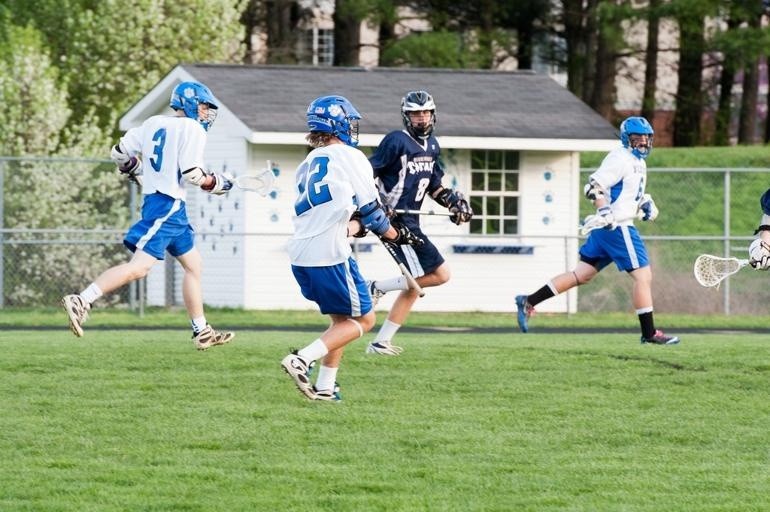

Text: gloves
xmin=200 ymin=170 xmax=236 ymax=197
xmin=596 ymin=205 xmax=619 ymax=232
xmin=350 ymin=210 xmax=369 ymax=239
xmin=379 ymin=192 xmax=397 ymax=222
xmin=638 ymin=193 xmax=659 ymax=222
xmin=118 ymin=156 xmax=144 ymax=188
xmin=433 ymin=187 xmax=474 ymax=225
xmin=393 ymin=221 xmax=425 ymax=248
xmin=748 ymin=240 xmax=770 ymax=272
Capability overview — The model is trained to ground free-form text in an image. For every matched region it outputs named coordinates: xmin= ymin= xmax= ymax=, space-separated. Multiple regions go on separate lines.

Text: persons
xmin=368 ymin=89 xmax=474 ymax=357
xmin=277 ymin=96 xmax=426 ymax=400
xmin=60 ymin=80 xmax=237 ymax=352
xmin=515 ymin=115 xmax=678 ymax=343
xmin=747 ymin=187 xmax=769 ymax=271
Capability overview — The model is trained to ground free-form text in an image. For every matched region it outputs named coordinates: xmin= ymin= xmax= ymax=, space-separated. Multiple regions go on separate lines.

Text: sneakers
xmin=515 ymin=295 xmax=535 ymax=333
xmin=640 ymin=329 xmax=681 ymax=345
xmin=365 ymin=341 xmax=404 ymax=357
xmin=366 ymin=279 xmax=384 ymax=308
xmin=61 ymin=293 xmax=92 ymax=337
xmin=190 ymin=324 xmax=235 ymax=352
xmin=311 ymin=380 xmax=344 ymax=404
xmin=280 ymin=349 xmax=319 ymax=401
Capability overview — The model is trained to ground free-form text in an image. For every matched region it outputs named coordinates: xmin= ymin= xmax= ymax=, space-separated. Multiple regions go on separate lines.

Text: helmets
xmin=169 ymin=80 xmax=219 ymax=132
xmin=619 ymin=116 xmax=655 ymax=159
xmin=399 ymin=89 xmax=438 ymax=141
xmin=306 ymin=95 xmax=362 ymax=149
